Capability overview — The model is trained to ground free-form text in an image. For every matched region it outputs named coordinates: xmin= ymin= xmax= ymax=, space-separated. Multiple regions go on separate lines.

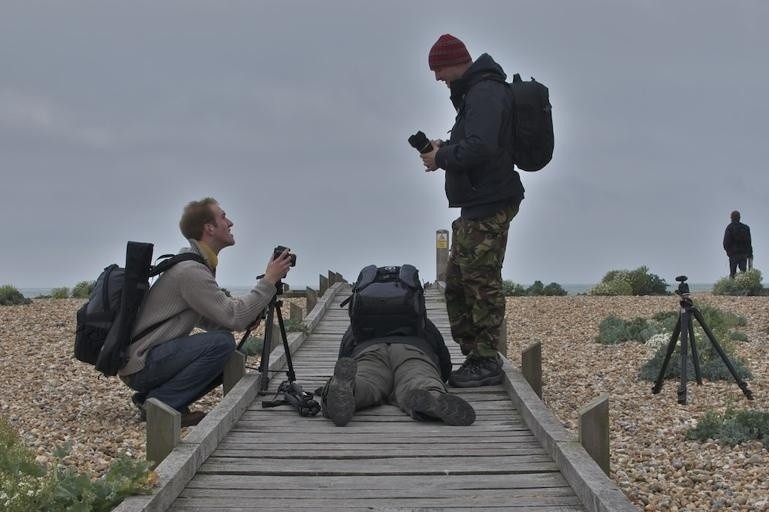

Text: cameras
xmin=273 ymin=244 xmax=296 ymax=267
xmin=408 ymin=131 xmax=451 ymax=156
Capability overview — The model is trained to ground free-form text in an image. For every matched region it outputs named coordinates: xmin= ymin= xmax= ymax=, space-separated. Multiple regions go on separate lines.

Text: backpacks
xmin=349 ymin=265 xmax=427 ymax=346
xmin=507 ymin=72 xmax=554 ymax=172
xmin=74 ymin=264 xmax=149 ymax=369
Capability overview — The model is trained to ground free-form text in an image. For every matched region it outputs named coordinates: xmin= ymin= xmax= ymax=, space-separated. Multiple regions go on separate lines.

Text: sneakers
xmin=449 ymin=359 xmax=507 ymax=387
xmin=404 ymin=389 xmax=476 ymax=426
xmin=130 ymin=392 xmax=206 ymax=426
xmin=326 ymin=358 xmax=356 ymax=427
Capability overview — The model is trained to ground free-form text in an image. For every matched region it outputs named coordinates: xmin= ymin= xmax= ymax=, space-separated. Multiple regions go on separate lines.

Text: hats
xmin=427 ymin=34 xmax=472 ymax=67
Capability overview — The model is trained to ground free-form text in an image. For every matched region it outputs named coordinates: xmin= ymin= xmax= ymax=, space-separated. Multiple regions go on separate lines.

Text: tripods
xmin=653 ymin=276 xmax=754 ymax=403
xmin=236 ymin=281 xmax=296 ymax=396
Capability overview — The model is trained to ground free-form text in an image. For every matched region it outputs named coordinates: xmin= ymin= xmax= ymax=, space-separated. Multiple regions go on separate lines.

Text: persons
xmin=722 ymin=210 xmax=753 ymax=279
xmin=318 ymin=320 xmax=476 ymax=427
xmin=418 ymin=32 xmax=526 ymax=389
xmin=110 ymin=195 xmax=293 ymax=428
